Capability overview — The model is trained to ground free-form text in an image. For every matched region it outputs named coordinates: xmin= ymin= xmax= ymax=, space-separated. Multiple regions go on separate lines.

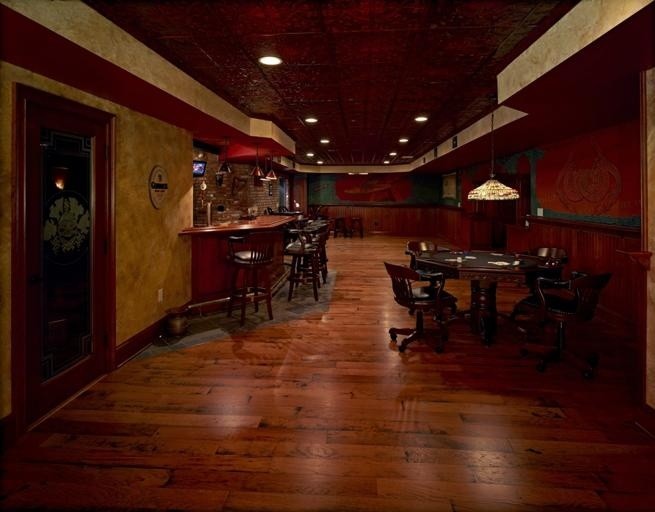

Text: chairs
xmin=224 ymin=206 xmax=334 ymax=328
xmin=382 ymin=238 xmax=613 ymax=380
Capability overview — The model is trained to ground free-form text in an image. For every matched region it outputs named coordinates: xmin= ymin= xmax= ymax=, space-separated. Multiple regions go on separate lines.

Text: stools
xmin=334 ymin=217 xmax=364 ymax=239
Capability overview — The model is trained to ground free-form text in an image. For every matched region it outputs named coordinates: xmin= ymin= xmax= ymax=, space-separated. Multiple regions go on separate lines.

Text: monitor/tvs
xmin=192 ymin=160 xmax=207 ymax=177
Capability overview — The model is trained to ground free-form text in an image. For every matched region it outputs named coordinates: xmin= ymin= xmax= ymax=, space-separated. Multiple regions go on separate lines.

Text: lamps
xmin=468 ymin=95 xmax=523 ymax=203
xmin=216 ymin=135 xmax=278 ymax=182
